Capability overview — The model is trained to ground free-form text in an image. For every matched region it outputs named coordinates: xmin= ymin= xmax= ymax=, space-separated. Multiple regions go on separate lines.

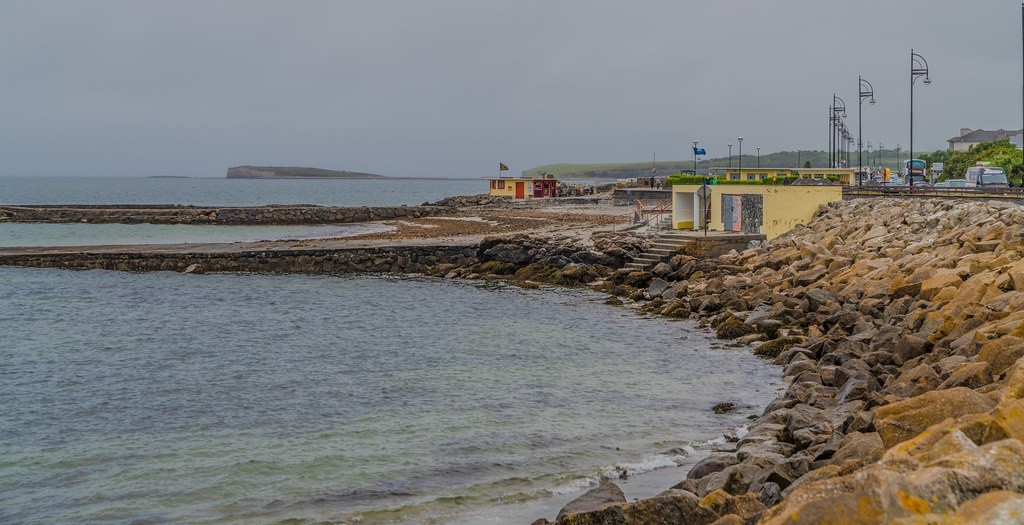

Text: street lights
xmin=728 ymin=144 xmax=732 ymax=168
xmin=796 ymin=149 xmax=801 ymax=170
xmin=738 ymin=137 xmax=744 ymax=180
xmin=858 ymin=75 xmax=876 ymax=189
xmin=828 ymin=104 xmax=855 ymax=168
xmin=910 ymin=48 xmax=936 ymax=192
xmin=693 ymin=140 xmax=698 ymax=175
xmin=833 ymin=92 xmax=847 ymax=168
xmin=756 ymin=146 xmax=760 ymax=168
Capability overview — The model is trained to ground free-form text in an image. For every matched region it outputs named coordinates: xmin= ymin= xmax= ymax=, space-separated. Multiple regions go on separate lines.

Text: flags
xmin=500 ymin=163 xmax=508 ymax=170
xmin=693 ymin=147 xmax=706 ymax=155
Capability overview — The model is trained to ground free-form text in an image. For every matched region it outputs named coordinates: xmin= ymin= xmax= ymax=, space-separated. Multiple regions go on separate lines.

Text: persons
xmin=649 ymin=176 xmax=661 ymax=189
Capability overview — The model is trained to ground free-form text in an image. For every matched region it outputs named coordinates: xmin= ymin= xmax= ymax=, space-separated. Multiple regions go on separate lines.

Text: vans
xmin=966 ymin=166 xmax=1010 ymax=194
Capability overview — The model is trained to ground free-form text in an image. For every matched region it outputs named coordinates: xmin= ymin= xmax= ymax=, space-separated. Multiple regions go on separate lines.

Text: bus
xmin=902 ymin=159 xmax=927 ymax=185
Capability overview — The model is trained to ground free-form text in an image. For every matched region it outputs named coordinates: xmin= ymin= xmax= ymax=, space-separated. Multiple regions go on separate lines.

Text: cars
xmin=864 ymin=172 xmax=945 ymax=193
xmin=944 ymin=178 xmax=967 ymax=193
